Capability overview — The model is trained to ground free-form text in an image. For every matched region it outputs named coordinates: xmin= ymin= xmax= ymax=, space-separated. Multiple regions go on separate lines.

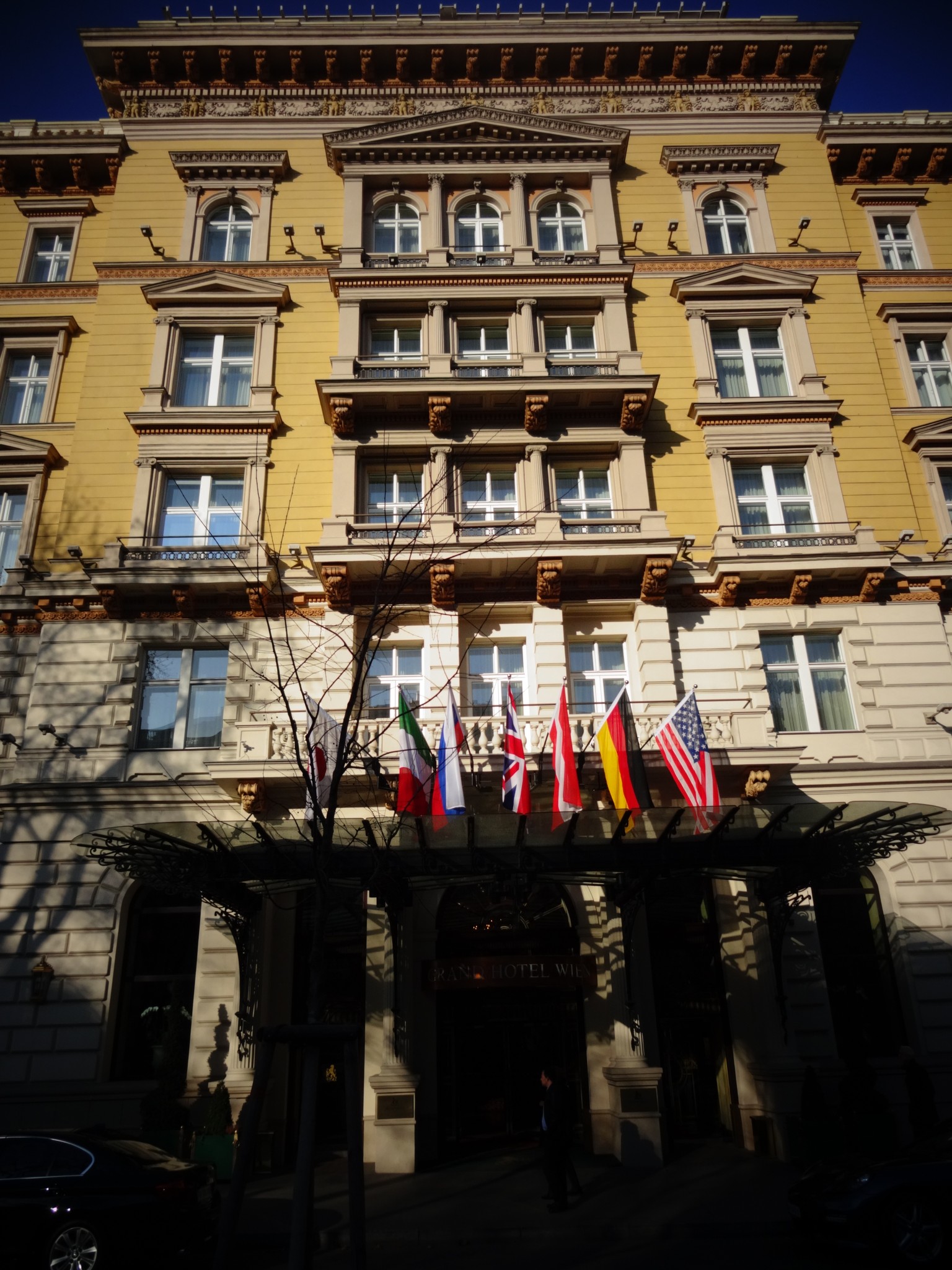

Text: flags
xmin=432 ymin=689 xmax=466 ymax=832
xmin=653 ymin=689 xmax=723 ymax=834
xmin=397 ymin=691 xmax=432 ymax=817
xmin=304 ymin=696 xmax=350 ymax=838
xmin=548 ymin=684 xmax=583 ymax=831
xmin=503 ymin=682 xmax=532 ymax=815
xmin=596 ymin=685 xmax=655 ymax=833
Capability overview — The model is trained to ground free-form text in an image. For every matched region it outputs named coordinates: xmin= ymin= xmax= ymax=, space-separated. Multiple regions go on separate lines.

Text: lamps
xmin=894 ymin=530 xmax=952 ymax=559
xmin=0 ymin=724 xmax=69 ymax=751
xmin=27 ymin=955 xmax=54 ymax=1007
xmin=681 ymin=535 xmax=696 ymax=557
xmin=141 ymin=218 xmax=812 ymax=265
xmin=18 ymin=545 xmax=87 ymax=581
xmin=288 ymin=544 xmax=302 ymax=560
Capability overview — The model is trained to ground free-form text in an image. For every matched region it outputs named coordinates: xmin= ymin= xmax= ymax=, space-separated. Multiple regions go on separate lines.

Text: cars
xmin=0 ymin=1132 xmax=203 ymax=1270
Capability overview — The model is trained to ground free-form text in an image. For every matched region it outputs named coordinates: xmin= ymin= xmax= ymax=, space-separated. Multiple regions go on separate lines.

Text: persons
xmin=540 ymin=1066 xmax=584 ymax=1213
